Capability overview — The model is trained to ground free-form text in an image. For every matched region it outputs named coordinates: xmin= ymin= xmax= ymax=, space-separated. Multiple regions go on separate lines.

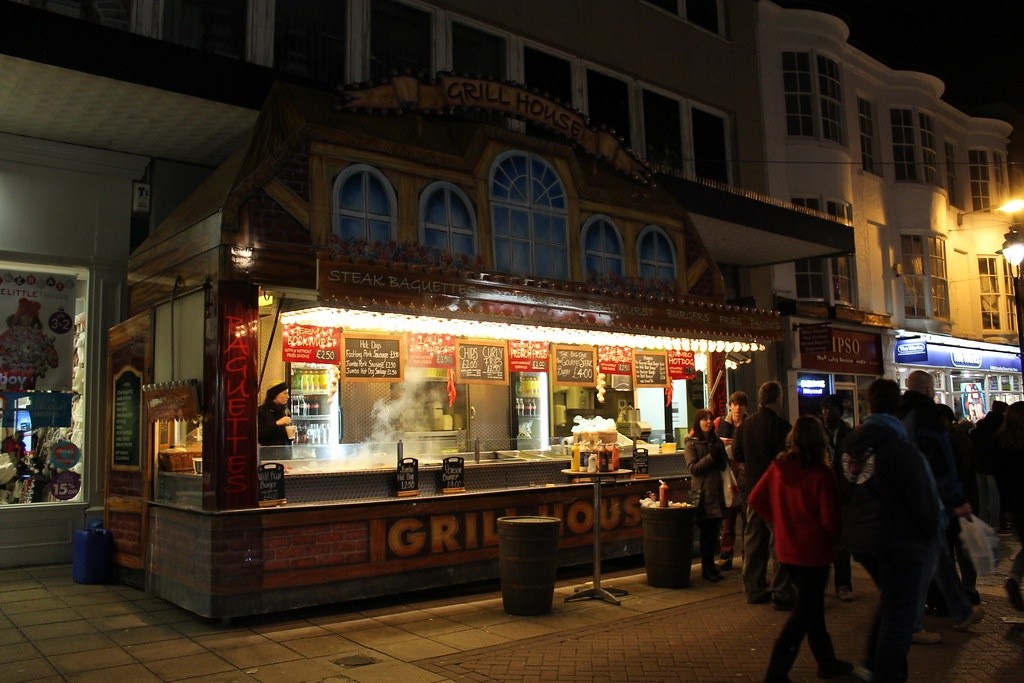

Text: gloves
xmin=710 ymin=446 xmax=722 ymax=459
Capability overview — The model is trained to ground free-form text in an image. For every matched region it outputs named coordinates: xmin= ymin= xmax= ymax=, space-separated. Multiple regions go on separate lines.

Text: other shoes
xmin=995 ymin=529 xmax=1012 ymax=537
xmin=817 ymin=660 xmax=854 ymax=679
xmin=837 ymin=589 xmax=852 ymax=600
xmin=912 ymin=631 xmax=942 ymax=643
xmin=953 ymin=606 xmax=985 ymax=630
xmin=703 ymin=569 xmax=724 ymax=583
xmin=719 ymin=560 xmax=733 ymax=571
xmin=1005 ymin=580 xmax=1024 ymax=611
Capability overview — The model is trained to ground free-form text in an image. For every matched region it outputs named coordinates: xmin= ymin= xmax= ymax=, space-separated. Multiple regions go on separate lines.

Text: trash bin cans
xmin=640 ymin=500 xmax=694 ymax=588
xmin=496 ymin=516 xmax=562 ymax=616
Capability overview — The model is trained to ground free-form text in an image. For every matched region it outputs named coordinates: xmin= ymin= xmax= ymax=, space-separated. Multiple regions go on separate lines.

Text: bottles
xmin=570 ymin=441 xmax=620 ymax=473
xmin=514 ymin=376 xmax=538 ymax=398
xmin=290 ymin=367 xmax=329 ymax=391
xmin=659 ymin=482 xmax=669 ymax=508
xmin=291 ymin=422 xmax=327 ymax=445
xmin=516 ymin=398 xmax=537 ymax=416
xmin=290 ymin=395 xmax=321 ymax=415
xmin=617 ymin=401 xmax=640 ymax=422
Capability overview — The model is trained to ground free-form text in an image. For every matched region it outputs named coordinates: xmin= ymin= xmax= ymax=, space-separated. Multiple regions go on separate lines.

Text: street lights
xmin=994 ymin=226 xmax=1024 ymax=393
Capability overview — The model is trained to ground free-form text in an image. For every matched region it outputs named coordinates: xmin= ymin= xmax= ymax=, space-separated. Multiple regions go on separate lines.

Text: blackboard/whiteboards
xmin=455 ymin=339 xmax=509 ymax=386
xmin=632 ymin=351 xmax=671 ymax=388
xmin=552 ymin=345 xmax=597 ymax=387
xmin=339 ymin=333 xmax=405 ymax=383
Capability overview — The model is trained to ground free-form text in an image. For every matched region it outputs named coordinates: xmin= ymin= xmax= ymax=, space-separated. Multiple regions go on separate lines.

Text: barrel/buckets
xmin=497 ymin=515 xmax=562 ymax=616
xmin=640 ymin=505 xmax=695 ymax=588
xmin=72 ymin=527 xmax=113 ymax=585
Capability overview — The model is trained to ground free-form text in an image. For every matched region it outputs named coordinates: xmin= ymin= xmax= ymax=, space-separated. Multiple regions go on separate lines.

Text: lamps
xmin=957 ymin=197 xmax=1024 ymax=226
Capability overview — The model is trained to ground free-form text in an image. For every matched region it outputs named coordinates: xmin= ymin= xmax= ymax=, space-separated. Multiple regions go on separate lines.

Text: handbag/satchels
xmin=958 ymin=513 xmax=1000 ymax=575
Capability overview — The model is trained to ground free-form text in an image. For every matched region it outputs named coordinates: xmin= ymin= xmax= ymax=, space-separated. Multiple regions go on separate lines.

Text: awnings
xmin=256 ymin=260 xmax=784 ymax=353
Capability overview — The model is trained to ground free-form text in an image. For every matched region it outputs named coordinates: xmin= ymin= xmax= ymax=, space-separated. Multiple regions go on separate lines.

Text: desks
xmin=499 ymin=515 xmax=561 ymax=615
xmin=641 ymin=505 xmax=696 ymax=591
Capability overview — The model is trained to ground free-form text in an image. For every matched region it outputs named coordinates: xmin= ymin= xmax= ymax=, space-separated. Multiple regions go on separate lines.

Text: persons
xmin=0 ymin=430 xmax=26 ymax=488
xmin=747 ymin=414 xmax=854 ymax=683
xmin=683 ymin=409 xmax=727 ymax=583
xmin=935 ymin=399 xmax=1024 ymax=617
xmin=685 ymin=392 xmax=750 ymax=570
xmin=731 ymin=382 xmax=797 ymax=610
xmin=813 ymin=395 xmax=853 ymax=604
xmin=832 ymin=379 xmax=949 ymax=683
xmin=894 ymin=371 xmax=980 ymax=643
xmin=257 ymin=382 xmax=298 ymax=467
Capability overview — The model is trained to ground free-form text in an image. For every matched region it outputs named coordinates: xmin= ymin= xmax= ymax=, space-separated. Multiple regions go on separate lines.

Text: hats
xmin=267 ymin=381 xmax=287 ymax=399
xmin=992 ymin=400 xmax=1008 ymax=414
xmin=820 ymin=394 xmax=844 ymax=416
